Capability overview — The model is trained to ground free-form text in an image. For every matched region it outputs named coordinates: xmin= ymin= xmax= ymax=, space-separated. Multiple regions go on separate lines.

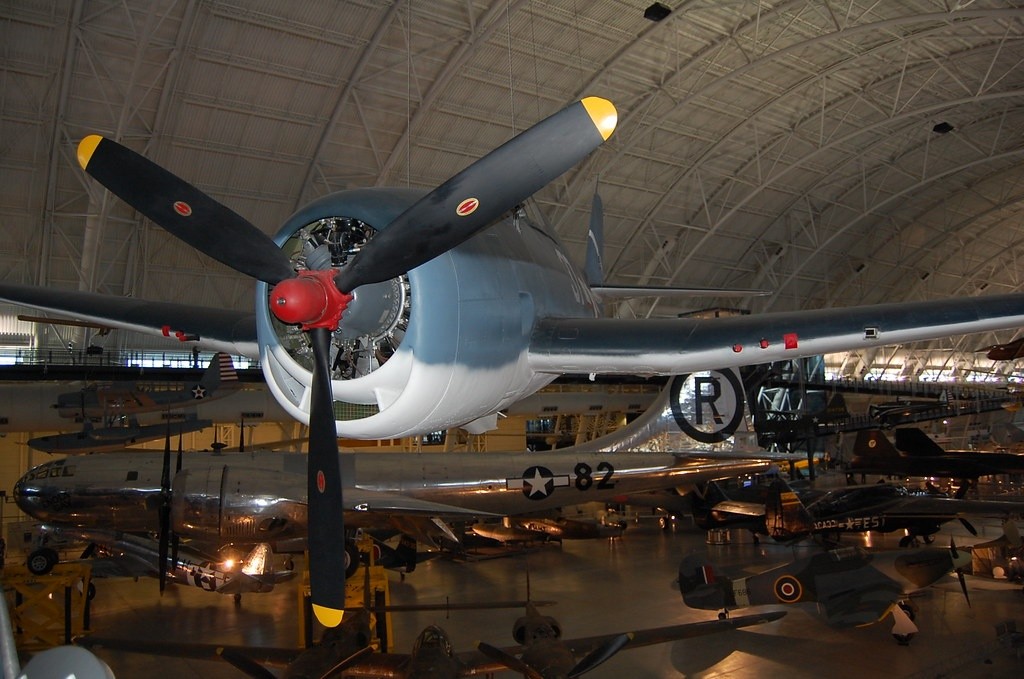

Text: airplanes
xmin=0 ymin=94 xmax=1023 ymax=679
